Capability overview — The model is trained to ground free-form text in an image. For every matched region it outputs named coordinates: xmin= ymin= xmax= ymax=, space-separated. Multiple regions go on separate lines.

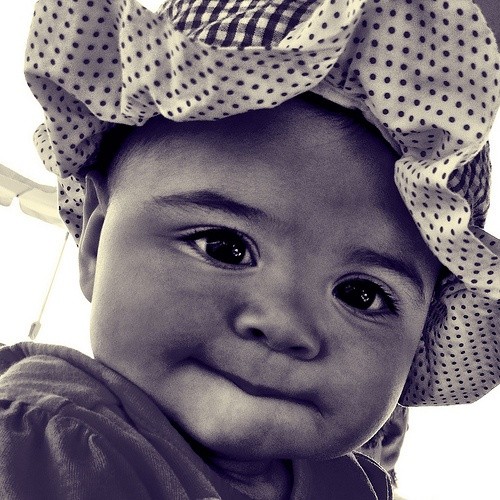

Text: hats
xmin=20 ymin=0 xmax=500 ymax=406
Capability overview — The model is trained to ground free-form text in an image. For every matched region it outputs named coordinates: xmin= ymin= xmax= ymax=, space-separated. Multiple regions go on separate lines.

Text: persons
xmin=0 ymin=10 xmax=489 ymax=500
